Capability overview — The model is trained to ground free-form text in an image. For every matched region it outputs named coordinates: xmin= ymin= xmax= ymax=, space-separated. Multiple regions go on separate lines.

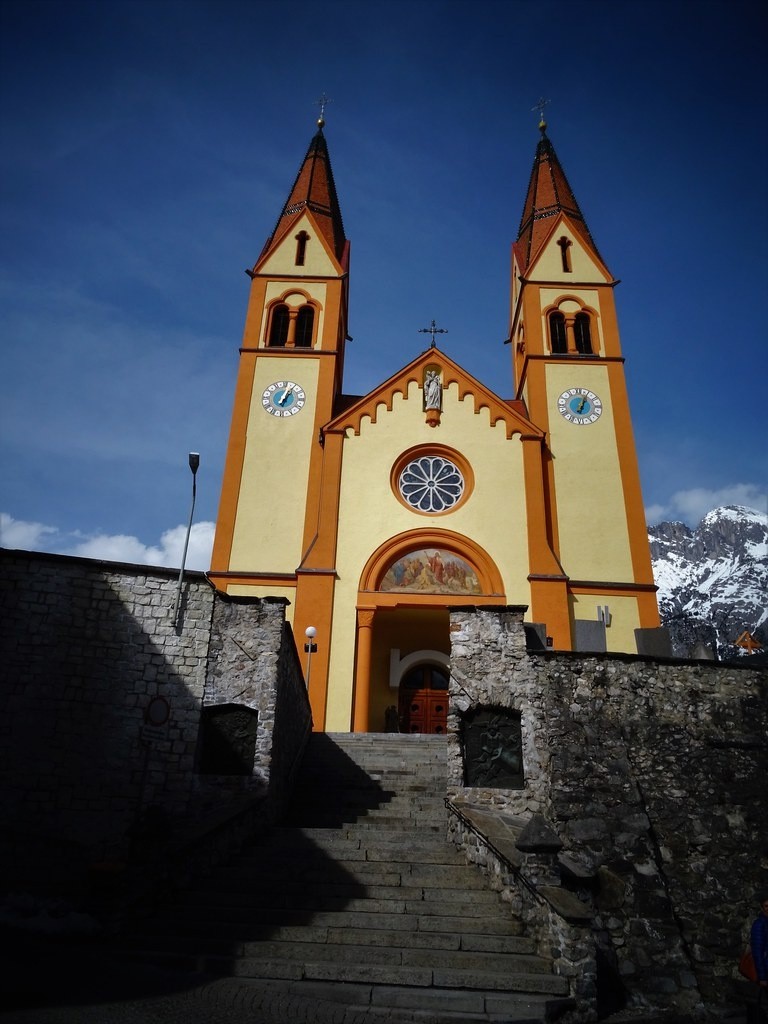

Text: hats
xmin=390 ymin=705 xmax=397 ymax=709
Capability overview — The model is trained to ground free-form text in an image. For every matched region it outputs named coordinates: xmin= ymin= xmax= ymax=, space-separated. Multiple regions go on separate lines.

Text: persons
xmin=425 ymin=369 xmax=441 ymax=410
xmin=384 ymin=704 xmax=400 ymax=734
